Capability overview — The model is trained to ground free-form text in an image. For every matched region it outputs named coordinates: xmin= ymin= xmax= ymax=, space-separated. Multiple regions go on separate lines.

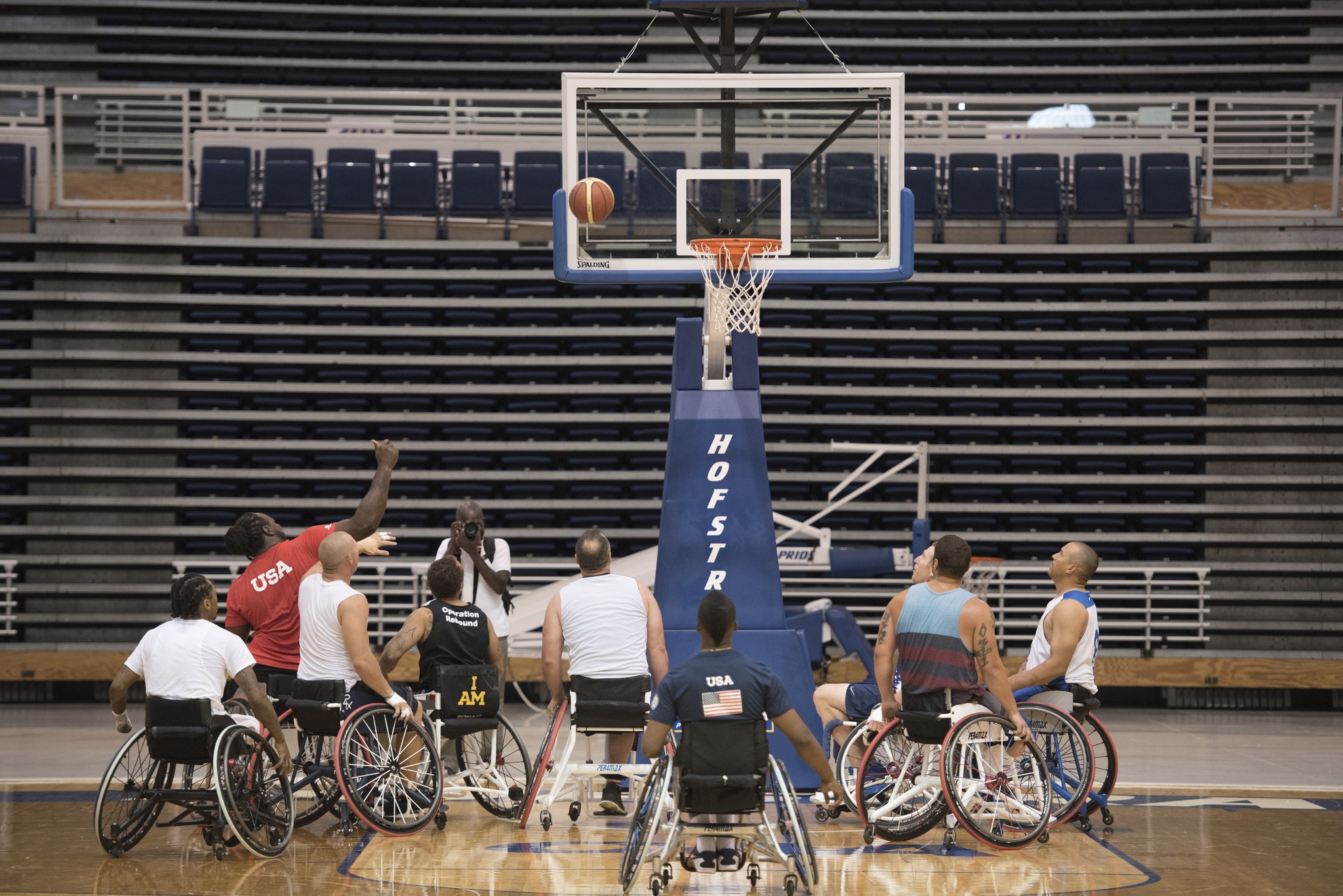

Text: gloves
xmin=113 ymin=711 xmax=132 ymax=734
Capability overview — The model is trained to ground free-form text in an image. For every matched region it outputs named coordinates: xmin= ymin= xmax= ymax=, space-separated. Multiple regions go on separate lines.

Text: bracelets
xmin=384 ymin=689 xmax=394 ymax=700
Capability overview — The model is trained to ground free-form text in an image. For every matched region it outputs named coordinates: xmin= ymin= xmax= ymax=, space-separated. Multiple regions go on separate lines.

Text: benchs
xmin=0 ymin=233 xmax=1343 ymax=657
xmin=2 ymin=0 xmax=1343 ymax=145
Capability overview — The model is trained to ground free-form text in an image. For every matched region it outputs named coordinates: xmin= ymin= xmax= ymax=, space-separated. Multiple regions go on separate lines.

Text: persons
xmin=225 ymin=439 xmax=669 ymax=814
xmin=641 ymin=589 xmax=844 ymax=875
xmin=109 ymin=575 xmax=291 ymax=811
xmin=810 ymin=531 xmax=1100 ymax=805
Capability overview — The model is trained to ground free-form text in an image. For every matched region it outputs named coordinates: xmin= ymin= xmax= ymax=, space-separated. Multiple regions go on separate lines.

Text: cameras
xmin=458 ymin=521 xmax=479 ymax=541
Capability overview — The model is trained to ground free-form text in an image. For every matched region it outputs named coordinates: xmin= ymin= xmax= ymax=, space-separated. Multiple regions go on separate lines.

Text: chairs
xmin=188 ymin=146 xmax=1209 ymax=245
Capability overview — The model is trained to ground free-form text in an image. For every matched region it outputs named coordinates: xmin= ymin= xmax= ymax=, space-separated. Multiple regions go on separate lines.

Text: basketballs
xmin=568 ymin=177 xmax=615 ymax=223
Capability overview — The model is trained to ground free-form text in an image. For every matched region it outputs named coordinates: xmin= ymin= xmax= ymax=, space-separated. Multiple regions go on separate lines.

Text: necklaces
xmin=701 ymin=647 xmax=733 ymax=652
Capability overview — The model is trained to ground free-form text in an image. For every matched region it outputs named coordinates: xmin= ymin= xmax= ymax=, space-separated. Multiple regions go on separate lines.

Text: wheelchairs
xmin=93 ymin=696 xmax=295 ymax=860
xmin=814 ymin=686 xmax=1119 ymax=851
xmin=178 ymin=675 xmax=448 ymax=837
xmin=618 ymin=725 xmax=820 ymax=896
xmin=514 ymin=687 xmax=690 ymax=833
xmin=418 ymin=663 xmax=533 ymax=818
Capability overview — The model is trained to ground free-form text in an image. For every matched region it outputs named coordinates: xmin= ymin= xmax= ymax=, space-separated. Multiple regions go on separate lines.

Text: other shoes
xmin=400 ymin=784 xmax=435 ymax=801
xmin=685 ymin=844 xmax=717 ymax=874
xmin=845 ymin=762 xmax=888 ymax=793
xmin=717 ymin=848 xmax=739 ymax=872
xmin=600 ymin=787 xmax=626 ymax=814
